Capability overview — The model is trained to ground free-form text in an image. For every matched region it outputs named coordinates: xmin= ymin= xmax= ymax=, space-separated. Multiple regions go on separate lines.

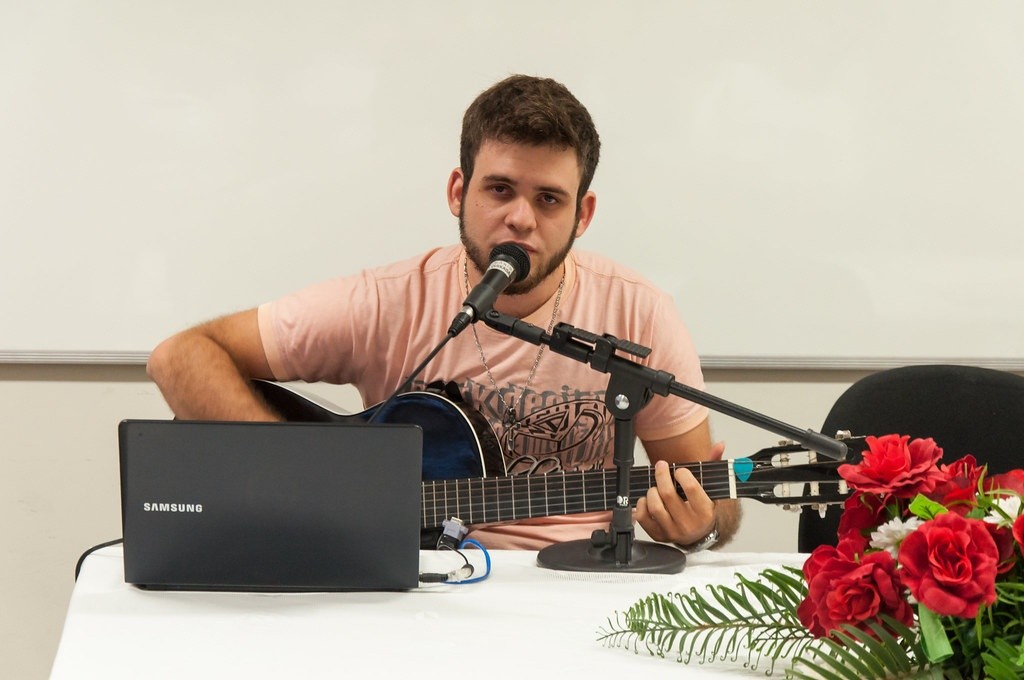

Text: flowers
xmin=595 ymin=433 xmax=1024 ymax=680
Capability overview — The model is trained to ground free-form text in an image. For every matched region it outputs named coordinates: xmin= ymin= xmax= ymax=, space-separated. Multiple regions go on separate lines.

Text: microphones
xmin=448 ymin=240 xmax=530 ymax=337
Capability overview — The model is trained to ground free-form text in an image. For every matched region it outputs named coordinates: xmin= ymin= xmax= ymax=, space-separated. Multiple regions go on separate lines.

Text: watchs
xmin=672 ymin=500 xmax=721 ymax=555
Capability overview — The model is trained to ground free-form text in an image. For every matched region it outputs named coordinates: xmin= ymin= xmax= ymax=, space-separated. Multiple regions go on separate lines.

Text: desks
xmin=49 ymin=542 xmax=912 ymax=680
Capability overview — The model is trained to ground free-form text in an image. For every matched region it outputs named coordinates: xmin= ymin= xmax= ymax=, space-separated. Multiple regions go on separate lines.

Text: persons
xmin=145 ymin=74 xmax=745 ymax=552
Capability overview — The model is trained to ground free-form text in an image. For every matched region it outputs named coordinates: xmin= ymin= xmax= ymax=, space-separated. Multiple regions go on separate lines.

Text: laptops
xmin=118 ymin=419 xmax=422 ymax=592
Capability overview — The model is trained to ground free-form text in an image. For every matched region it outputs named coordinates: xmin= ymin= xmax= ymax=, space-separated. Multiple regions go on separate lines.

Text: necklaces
xmin=463 ymin=252 xmax=566 ymax=429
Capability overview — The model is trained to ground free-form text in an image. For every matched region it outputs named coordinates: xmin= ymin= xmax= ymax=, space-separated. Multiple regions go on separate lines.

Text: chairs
xmin=796 ymin=366 xmax=1024 ymax=569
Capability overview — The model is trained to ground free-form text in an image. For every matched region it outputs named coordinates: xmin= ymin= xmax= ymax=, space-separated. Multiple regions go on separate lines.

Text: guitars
xmin=169 ymin=375 xmax=867 ymax=522
